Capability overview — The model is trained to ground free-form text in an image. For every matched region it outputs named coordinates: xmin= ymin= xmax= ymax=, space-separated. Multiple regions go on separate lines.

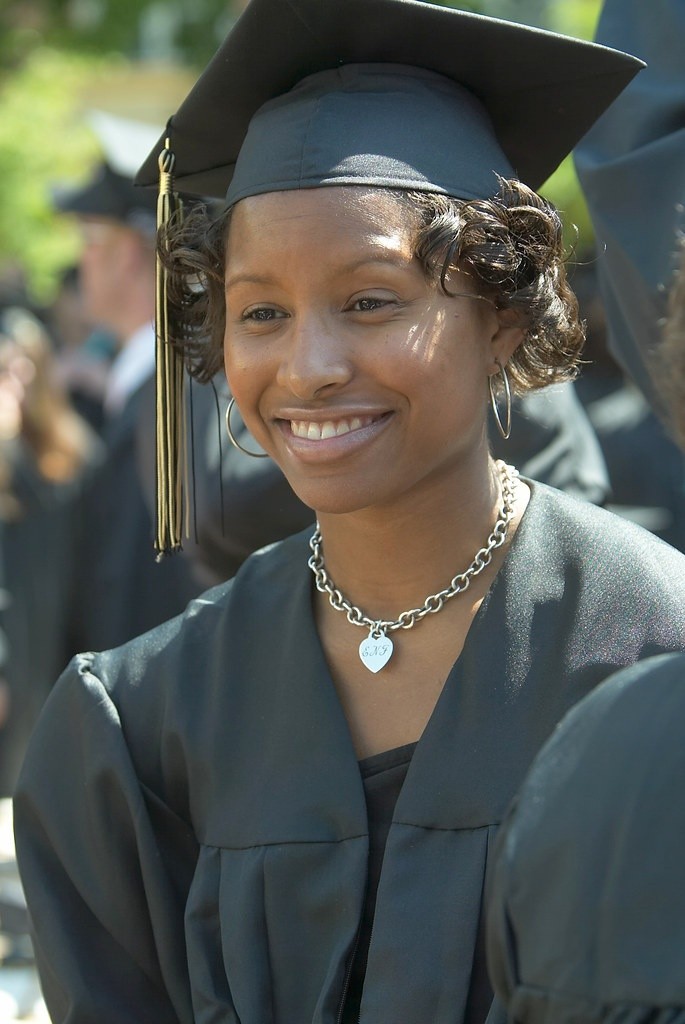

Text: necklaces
xmin=307 ymin=456 xmax=520 ymax=673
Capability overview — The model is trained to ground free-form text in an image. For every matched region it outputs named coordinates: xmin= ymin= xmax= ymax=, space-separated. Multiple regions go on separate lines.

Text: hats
xmin=53 ymin=112 xmax=159 ymax=220
xmin=132 ymin=0 xmax=646 ymax=557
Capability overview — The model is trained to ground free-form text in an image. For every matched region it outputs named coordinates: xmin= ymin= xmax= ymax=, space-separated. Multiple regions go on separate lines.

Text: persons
xmin=0 ymin=0 xmax=685 ymax=1024
